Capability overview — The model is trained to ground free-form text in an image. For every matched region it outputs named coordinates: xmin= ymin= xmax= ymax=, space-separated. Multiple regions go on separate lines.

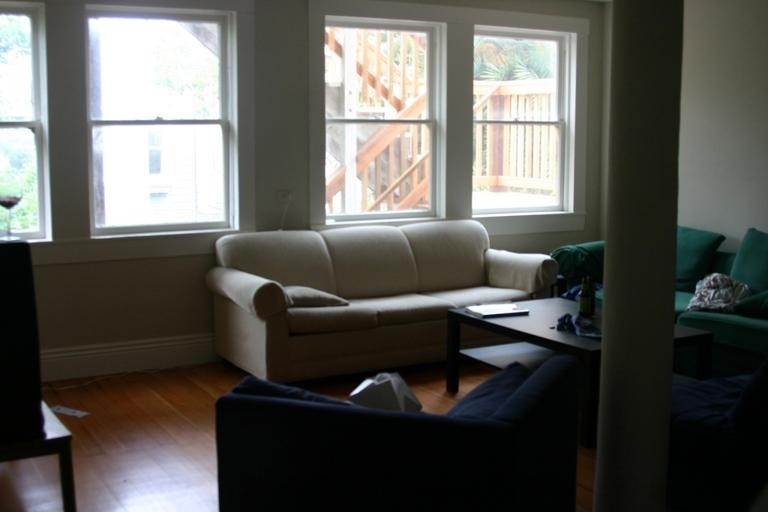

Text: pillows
xmin=284 ymin=284 xmax=349 ymax=309
xmin=674 ymin=225 xmax=726 ymax=291
xmin=730 ymin=227 xmax=768 ymax=293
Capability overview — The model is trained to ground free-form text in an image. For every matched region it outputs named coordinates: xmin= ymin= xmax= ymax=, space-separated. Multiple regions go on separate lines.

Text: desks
xmin=0 ymin=396 xmax=78 ymax=512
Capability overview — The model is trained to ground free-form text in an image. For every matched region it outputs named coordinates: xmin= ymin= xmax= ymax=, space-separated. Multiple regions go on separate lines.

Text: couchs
xmin=551 ymin=234 xmax=768 ymax=317
xmin=205 ymin=218 xmax=559 ymax=388
xmin=214 ymin=352 xmax=580 ymax=512
xmin=667 ymin=308 xmax=768 ymax=512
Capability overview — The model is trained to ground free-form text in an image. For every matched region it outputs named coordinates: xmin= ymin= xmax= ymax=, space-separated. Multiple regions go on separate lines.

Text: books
xmin=464 ymin=302 xmax=530 ymax=318
